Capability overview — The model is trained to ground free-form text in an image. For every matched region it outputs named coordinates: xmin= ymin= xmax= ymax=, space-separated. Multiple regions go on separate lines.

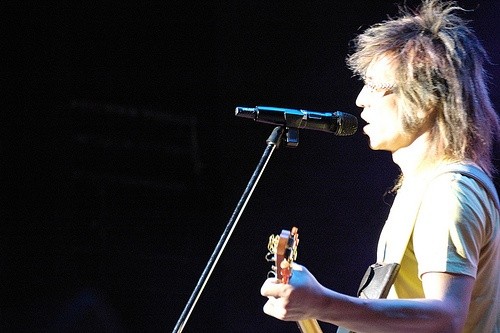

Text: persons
xmin=260 ymin=11 xmax=500 ymax=333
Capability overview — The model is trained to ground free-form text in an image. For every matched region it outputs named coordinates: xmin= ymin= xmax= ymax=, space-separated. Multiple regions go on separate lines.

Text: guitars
xmin=265 ymin=226 xmax=322 ymax=333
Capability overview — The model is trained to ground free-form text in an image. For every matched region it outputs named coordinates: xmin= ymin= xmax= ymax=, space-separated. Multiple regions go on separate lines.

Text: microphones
xmin=235 ymin=103 xmax=358 ymax=139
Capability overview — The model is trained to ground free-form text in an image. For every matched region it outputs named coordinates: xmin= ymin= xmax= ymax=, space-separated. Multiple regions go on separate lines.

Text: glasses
xmin=363 ymin=78 xmax=403 ymax=95
